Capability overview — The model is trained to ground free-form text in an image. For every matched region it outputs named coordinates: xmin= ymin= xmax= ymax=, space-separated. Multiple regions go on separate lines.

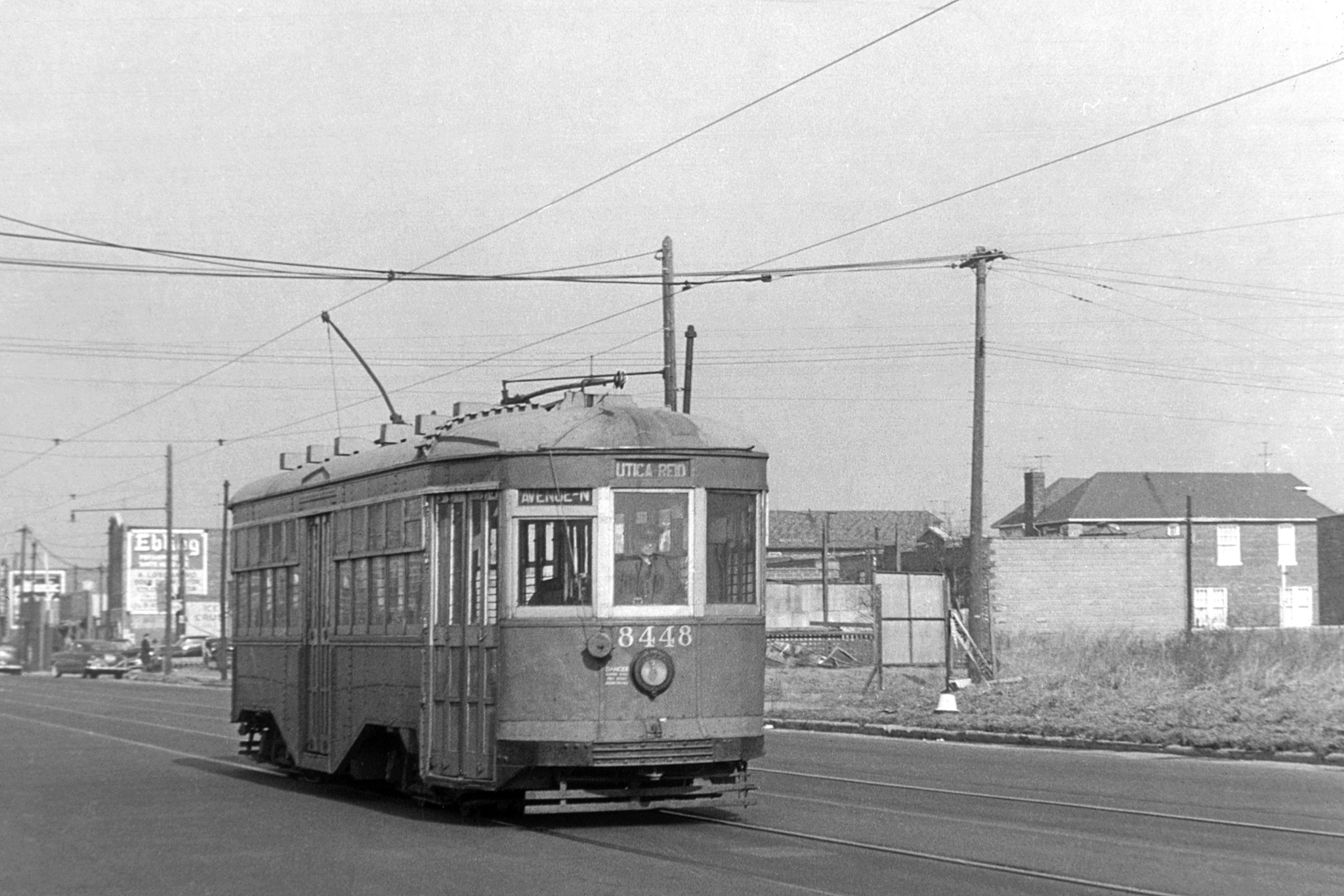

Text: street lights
xmin=69 ymin=506 xmax=172 ymax=676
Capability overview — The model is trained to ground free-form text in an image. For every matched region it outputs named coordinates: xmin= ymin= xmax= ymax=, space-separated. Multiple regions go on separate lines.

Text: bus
xmin=226 ymin=311 xmax=771 ymax=826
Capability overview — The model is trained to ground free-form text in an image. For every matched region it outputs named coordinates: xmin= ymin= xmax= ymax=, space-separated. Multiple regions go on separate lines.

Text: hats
xmin=632 ymin=523 xmax=664 ymax=541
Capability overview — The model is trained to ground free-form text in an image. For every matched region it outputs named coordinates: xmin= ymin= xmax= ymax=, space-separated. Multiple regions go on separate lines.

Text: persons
xmin=614 ymin=522 xmax=686 ymax=605
xmin=140 ymin=633 xmax=152 ymax=665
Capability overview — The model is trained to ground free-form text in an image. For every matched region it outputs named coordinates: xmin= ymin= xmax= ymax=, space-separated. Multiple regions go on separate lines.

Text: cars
xmin=157 ymin=635 xmax=210 ymax=658
xmin=0 ymin=645 xmax=23 ymax=676
xmin=50 ymin=638 xmax=132 ymax=679
xmin=201 ymin=637 xmax=235 ymax=668
xmin=111 ymin=638 xmax=142 ymax=660
